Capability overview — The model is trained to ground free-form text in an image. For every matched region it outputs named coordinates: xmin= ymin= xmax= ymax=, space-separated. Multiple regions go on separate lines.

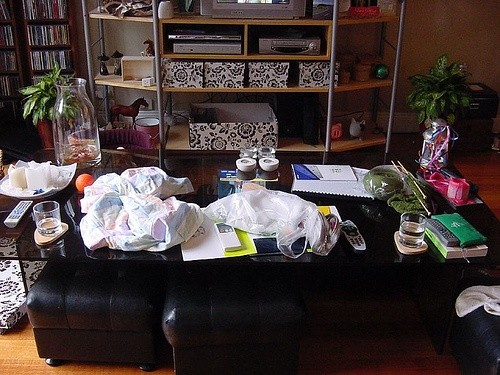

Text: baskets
xmin=416 ymin=164 xmax=485 ymax=236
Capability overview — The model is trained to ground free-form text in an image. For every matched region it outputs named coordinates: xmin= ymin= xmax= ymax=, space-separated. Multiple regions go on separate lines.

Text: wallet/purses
xmin=431 ymin=212 xmax=488 ymax=264
xmin=301 ymin=209 xmax=341 ymax=256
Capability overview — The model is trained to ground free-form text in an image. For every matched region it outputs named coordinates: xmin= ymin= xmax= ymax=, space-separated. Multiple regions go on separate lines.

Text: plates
xmin=0 ymin=160 xmax=78 ymax=200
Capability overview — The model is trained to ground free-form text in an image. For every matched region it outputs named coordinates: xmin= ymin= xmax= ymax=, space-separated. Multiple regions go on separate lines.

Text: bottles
xmin=52 ymin=77 xmax=103 ymax=170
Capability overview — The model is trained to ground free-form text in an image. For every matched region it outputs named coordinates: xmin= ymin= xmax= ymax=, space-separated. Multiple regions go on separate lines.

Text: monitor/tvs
xmin=199 ymin=0 xmax=314 ymax=20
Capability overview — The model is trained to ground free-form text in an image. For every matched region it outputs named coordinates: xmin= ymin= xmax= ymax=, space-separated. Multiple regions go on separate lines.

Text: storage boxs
xmin=298 ymin=61 xmax=339 ymax=88
xmin=187 ymin=102 xmax=279 ymax=150
xmin=162 ymin=60 xmax=203 ymax=88
xmin=247 ymin=61 xmax=290 ymax=88
xmin=205 ymin=60 xmax=245 ymax=87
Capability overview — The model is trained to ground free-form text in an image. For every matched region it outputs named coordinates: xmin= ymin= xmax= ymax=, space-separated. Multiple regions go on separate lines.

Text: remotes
xmin=4 ymin=201 xmax=34 ymax=229
xmin=339 ymin=219 xmax=367 ymax=255
xmin=214 ymin=222 xmax=242 ymax=251
xmin=427 ymin=218 xmax=460 ymax=247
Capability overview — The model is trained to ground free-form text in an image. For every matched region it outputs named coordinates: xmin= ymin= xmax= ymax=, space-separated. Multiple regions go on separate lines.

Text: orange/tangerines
xmin=76 ymin=174 xmax=94 ymax=192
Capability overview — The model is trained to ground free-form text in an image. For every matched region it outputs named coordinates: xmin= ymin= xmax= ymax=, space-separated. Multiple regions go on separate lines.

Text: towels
xmin=454 ymin=283 xmax=500 ymax=319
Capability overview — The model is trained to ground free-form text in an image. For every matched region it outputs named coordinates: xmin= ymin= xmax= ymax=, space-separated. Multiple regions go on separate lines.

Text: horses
xmin=143 ymin=39 xmax=156 ymax=57
xmin=109 ymin=96 xmax=149 ymax=128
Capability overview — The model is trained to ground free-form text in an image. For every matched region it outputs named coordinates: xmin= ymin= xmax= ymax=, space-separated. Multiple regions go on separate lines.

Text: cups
xmin=398 ymin=211 xmax=428 ymax=249
xmin=135 ymin=117 xmax=160 ymax=145
xmin=33 ymin=200 xmax=63 ymax=236
xmin=40 ymin=239 xmax=67 ymax=259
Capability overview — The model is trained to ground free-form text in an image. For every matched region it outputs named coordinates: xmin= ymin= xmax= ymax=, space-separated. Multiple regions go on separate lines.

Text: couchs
xmin=450 ymin=290 xmax=500 ymax=375
xmin=26 ymin=260 xmax=160 ymax=372
xmin=162 ymin=264 xmax=306 ymax=374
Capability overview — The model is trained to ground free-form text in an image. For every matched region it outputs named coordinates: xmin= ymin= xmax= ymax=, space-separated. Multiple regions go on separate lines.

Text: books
xmin=34 ymin=74 xmax=73 ymax=85
xmin=0 ymin=1 xmax=11 ymax=20
xmin=27 ymin=24 xmax=71 ymax=46
xmin=30 ymin=49 xmax=72 ymax=70
xmin=425 ymin=227 xmax=488 ymax=260
xmin=22 ymin=0 xmax=67 ymax=21
xmin=0 ymin=99 xmax=24 ymax=121
xmin=0 ymin=51 xmax=17 ymax=72
xmin=0 ymin=25 xmax=15 ymax=46
xmin=291 ymin=163 xmax=358 ymax=183
xmin=247 ymin=231 xmax=305 ymax=258
xmin=0 ymin=75 xmax=21 ymax=97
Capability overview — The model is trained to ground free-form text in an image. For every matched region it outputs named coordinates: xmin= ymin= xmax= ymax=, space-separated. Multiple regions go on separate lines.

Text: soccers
xmin=375 ymin=64 xmax=389 ymax=79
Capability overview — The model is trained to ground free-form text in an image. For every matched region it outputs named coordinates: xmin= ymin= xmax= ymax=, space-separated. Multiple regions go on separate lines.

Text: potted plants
xmin=406 ymin=52 xmax=475 ymax=140
xmin=16 ymin=61 xmax=82 ymax=150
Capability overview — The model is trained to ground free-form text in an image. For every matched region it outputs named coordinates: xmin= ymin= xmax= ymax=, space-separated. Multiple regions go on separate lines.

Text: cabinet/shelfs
xmin=80 ymin=0 xmax=406 ymax=153
xmin=2 ymin=0 xmax=41 ymax=162
xmin=20 ymin=0 xmax=78 ymax=149
